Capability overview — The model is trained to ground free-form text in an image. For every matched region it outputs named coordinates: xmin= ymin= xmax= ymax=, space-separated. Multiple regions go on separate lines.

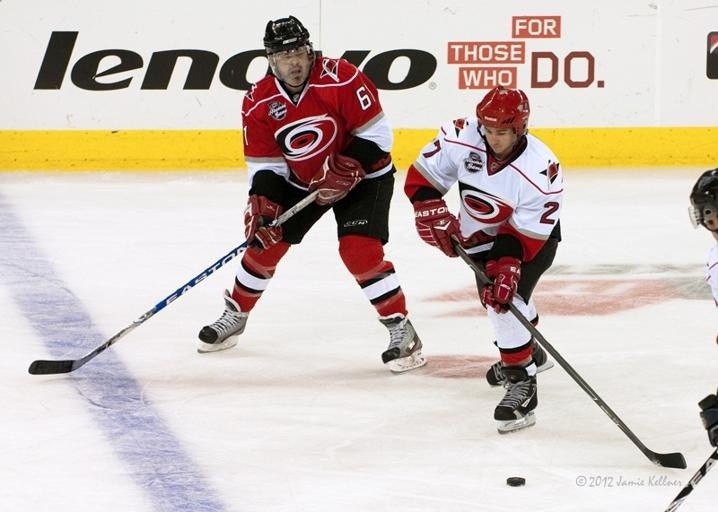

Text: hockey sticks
xmin=27 ymin=186 xmax=320 ymax=375
xmin=446 ymin=233 xmax=687 ymax=470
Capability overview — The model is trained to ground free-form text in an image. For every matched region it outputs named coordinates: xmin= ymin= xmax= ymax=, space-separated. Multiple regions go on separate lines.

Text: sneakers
xmin=486 ymin=342 xmax=548 ymax=386
xmin=194 ymin=288 xmax=248 ymax=345
xmin=377 ymin=313 xmax=423 ymax=363
xmin=492 ymin=360 xmax=539 ymax=421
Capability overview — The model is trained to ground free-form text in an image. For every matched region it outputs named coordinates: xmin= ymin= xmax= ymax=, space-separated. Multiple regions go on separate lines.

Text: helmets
xmin=687 ymin=167 xmax=718 ymax=233
xmin=262 ymin=15 xmax=310 ymax=50
xmin=475 ymin=84 xmax=530 ymax=140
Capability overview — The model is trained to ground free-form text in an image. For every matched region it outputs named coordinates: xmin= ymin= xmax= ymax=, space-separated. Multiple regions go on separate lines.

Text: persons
xmin=403 ymin=84 xmax=564 ymax=426
xmin=199 ymin=13 xmax=424 ymax=380
xmin=686 ymin=167 xmax=718 ymax=450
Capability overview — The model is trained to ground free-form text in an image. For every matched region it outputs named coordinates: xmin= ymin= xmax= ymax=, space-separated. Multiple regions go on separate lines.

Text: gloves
xmin=308 ymin=153 xmax=366 ymax=207
xmin=697 ymin=393 xmax=718 ymax=447
xmin=242 ymin=192 xmax=284 ymax=255
xmin=479 ymin=254 xmax=523 ymax=315
xmin=413 ymin=198 xmax=465 ymax=258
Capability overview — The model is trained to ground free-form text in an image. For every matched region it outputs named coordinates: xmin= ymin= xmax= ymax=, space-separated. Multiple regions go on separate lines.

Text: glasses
xmin=277 ymin=45 xmax=310 ymax=59
